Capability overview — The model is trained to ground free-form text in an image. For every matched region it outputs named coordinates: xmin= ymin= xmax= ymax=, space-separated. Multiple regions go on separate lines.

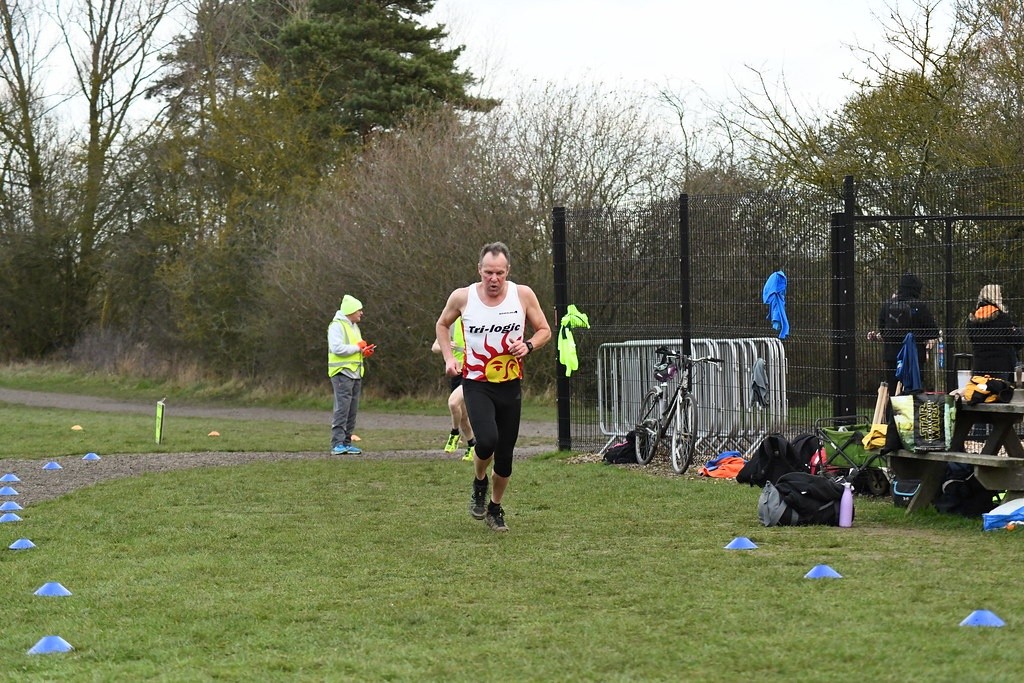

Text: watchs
xmin=524 ymin=341 xmax=533 ymax=354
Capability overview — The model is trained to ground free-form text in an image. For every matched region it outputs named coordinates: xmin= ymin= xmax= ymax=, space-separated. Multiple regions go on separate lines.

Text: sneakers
xmin=330 ymin=444 xmax=348 ymax=454
xmin=470 ymin=482 xmax=488 ymax=520
xmin=445 ymin=432 xmax=460 ymax=454
xmin=483 ymin=508 xmax=510 ymax=532
xmin=344 ymin=445 xmax=362 ymax=455
xmin=463 ymin=444 xmax=479 ymax=461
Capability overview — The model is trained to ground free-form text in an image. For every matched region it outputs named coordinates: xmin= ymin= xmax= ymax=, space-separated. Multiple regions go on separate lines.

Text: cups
xmin=957 ymin=370 xmax=972 ymax=402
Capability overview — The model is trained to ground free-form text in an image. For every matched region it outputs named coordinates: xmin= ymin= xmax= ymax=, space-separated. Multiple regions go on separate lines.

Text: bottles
xmin=839 ymin=482 xmax=853 ymax=527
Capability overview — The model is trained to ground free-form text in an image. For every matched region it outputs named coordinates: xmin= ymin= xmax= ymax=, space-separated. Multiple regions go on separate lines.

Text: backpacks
xmin=737 ymin=433 xmax=839 ymax=486
xmin=605 ymin=430 xmax=654 ymax=464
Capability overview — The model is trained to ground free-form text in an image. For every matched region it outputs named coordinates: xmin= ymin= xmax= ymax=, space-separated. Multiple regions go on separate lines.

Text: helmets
xmin=652 ymin=361 xmax=676 ymax=381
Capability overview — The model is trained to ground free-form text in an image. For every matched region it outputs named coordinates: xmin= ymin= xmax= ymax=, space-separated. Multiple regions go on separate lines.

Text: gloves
xmin=358 ymin=340 xmax=375 ymax=358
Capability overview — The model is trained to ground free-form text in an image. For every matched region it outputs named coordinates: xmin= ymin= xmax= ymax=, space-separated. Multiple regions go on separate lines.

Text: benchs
xmin=866 ymin=445 xmax=1024 ymax=491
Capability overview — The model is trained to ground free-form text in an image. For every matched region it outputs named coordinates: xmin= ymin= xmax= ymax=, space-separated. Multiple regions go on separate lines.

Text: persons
xmin=878 ymin=272 xmax=940 ymax=397
xmin=325 ymin=295 xmax=374 ymax=456
xmin=966 ymin=282 xmax=1024 ymax=393
xmin=435 ymin=242 xmax=552 ymax=534
xmin=430 ymin=313 xmax=476 ymax=462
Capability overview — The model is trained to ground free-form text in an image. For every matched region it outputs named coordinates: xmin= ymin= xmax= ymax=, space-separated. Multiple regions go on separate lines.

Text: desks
xmin=905 ymin=401 xmax=1024 ymax=517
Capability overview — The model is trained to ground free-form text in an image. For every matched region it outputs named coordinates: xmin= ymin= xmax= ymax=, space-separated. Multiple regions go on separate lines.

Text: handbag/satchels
xmin=892 ymin=482 xmax=919 ymax=508
xmin=965 ymin=376 xmax=1015 ymax=404
xmin=757 ymin=472 xmax=857 ymax=527
xmin=933 ymin=461 xmax=995 ymax=518
xmin=862 ymin=383 xmax=952 ymax=454
xmin=835 ymin=467 xmax=890 ymax=496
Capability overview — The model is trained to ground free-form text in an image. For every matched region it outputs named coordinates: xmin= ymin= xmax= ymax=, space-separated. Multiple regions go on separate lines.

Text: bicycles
xmin=633 ymin=344 xmax=725 ymax=475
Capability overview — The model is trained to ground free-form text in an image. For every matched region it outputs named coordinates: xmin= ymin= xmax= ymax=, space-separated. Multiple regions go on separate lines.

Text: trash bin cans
xmin=954 ymin=352 xmax=973 ymax=389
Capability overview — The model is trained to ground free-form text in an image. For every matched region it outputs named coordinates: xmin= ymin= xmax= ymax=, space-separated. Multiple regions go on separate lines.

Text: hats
xmin=976 ymin=284 xmax=1008 ymax=314
xmin=900 ymin=273 xmax=922 ymax=291
xmin=339 ymin=295 xmax=362 ymax=315
